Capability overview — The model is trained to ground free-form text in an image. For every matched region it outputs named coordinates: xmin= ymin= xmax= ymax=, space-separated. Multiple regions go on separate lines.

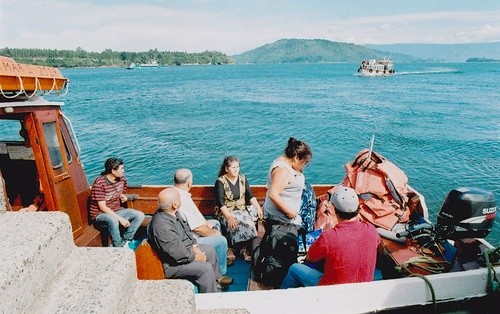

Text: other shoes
xmin=218 ymin=276 xmax=233 ymax=285
xmin=227 ymin=248 xmax=235 ymax=265
xmin=240 ymin=249 xmax=251 ymax=262
xmin=217 ymin=283 xmax=229 ymax=293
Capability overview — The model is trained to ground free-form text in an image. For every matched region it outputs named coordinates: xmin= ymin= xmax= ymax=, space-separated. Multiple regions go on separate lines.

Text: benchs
xmin=135 ymin=238 xmax=198 ymax=294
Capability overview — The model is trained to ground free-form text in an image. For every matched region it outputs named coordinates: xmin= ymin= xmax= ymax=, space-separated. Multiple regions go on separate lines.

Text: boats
xmin=354 ymin=59 xmax=396 ymax=77
xmin=0 ymin=56 xmax=500 ymax=313
xmin=140 ymin=57 xmax=159 ymax=67
xmin=126 ymin=65 xmax=138 ymax=70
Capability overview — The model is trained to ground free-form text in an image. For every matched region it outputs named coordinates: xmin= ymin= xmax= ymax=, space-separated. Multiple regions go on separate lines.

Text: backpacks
xmin=251 ymin=222 xmax=307 ymax=288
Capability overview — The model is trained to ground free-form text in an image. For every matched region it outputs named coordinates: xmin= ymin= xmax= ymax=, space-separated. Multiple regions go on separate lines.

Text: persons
xmin=280 ymin=187 xmax=381 ymax=289
xmin=88 ymin=157 xmax=146 ymax=247
xmin=147 ymin=137 xmax=312 ymax=293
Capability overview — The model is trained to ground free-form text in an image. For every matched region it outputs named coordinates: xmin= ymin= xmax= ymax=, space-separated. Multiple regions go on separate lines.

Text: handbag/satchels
xmin=247 ymin=205 xmax=259 ymax=222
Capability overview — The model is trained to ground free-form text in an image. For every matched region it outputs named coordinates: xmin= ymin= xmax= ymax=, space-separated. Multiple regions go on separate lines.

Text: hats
xmin=330 ymin=188 xmax=359 ymax=212
